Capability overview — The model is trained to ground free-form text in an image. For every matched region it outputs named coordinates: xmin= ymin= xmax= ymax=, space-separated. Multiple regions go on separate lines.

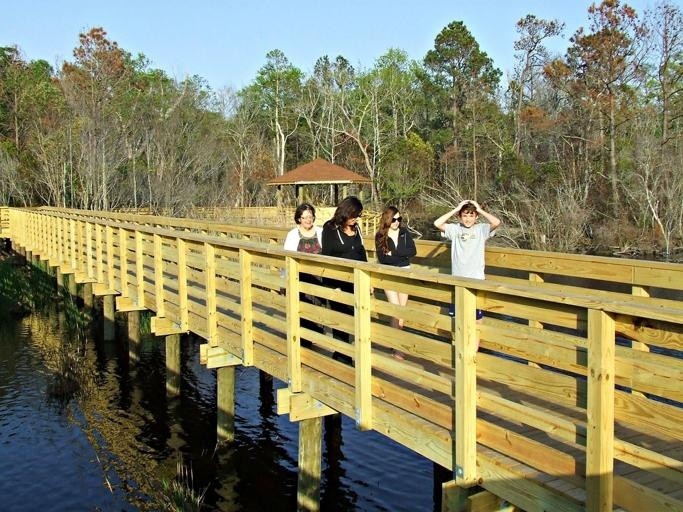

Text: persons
xmin=433 ymin=199 xmax=501 ymax=351
xmin=375 ymin=206 xmax=418 ymax=360
xmin=320 ymin=195 xmax=374 ymax=366
xmin=280 ymin=203 xmax=324 ymax=348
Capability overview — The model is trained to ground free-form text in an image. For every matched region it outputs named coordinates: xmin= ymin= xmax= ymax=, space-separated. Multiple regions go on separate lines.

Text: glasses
xmin=352 ymin=212 xmax=362 ymax=218
xmin=392 ymin=217 xmax=403 ymax=222
xmin=300 ymin=216 xmax=315 ymax=221
